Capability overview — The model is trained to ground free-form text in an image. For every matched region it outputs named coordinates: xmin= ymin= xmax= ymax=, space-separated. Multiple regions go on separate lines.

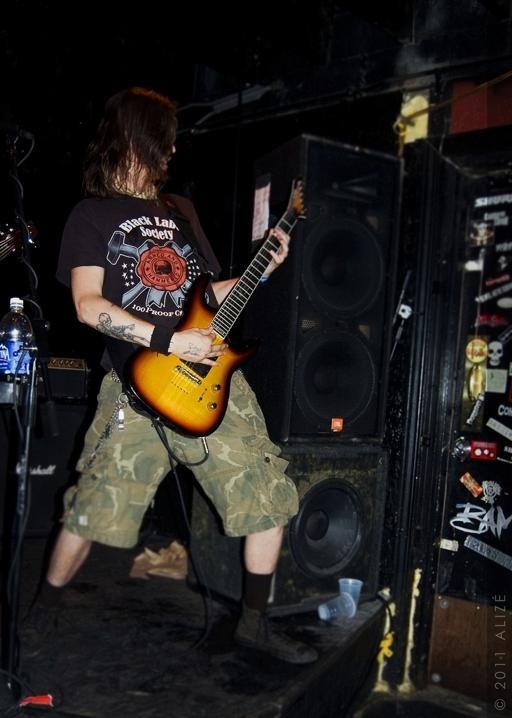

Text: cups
xmin=337 ymin=578 xmax=364 ymax=605
xmin=317 ymin=591 xmax=356 ymax=621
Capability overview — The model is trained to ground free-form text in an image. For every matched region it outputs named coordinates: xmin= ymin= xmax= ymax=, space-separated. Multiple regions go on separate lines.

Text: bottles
xmin=0 ymin=296 xmax=35 ymax=386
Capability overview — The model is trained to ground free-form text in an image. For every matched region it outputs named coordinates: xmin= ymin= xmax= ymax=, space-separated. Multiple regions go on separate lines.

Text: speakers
xmin=10 ymin=404 xmax=99 ymax=537
xmin=227 ymin=133 xmax=404 ymax=445
xmin=186 ymin=443 xmax=389 ymax=618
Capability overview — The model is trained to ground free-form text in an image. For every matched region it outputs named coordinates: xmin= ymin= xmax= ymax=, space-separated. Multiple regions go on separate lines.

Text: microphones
xmin=39 ymin=357 xmax=59 ymax=439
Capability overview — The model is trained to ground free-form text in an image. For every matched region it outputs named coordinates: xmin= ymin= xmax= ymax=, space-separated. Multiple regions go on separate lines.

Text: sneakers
xmin=233 ymin=599 xmax=318 ymax=663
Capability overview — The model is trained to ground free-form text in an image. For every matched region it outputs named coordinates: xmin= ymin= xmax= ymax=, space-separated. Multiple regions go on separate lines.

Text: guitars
xmin=121 ymin=172 xmax=307 ymax=438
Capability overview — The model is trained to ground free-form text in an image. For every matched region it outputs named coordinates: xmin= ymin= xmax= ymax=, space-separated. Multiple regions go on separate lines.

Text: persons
xmin=16 ymin=88 xmax=320 ymax=666
